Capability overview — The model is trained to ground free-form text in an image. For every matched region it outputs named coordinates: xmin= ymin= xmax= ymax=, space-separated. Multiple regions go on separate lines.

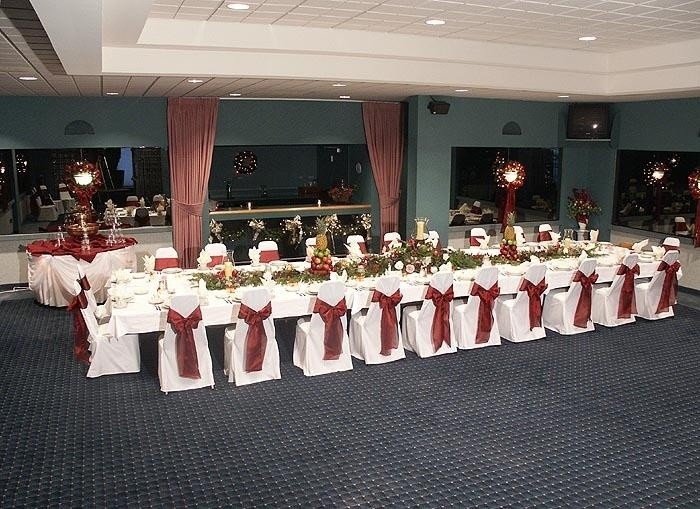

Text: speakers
xmin=428 ymin=101 xmax=450 ymax=115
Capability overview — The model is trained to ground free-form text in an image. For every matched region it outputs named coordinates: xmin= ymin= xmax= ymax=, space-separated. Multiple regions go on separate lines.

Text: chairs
xmin=73 ymin=280 xmax=142 ymax=378
xmin=89 ymin=195 xmax=172 ymax=227
xmin=674 ymin=216 xmax=695 ymax=236
xmin=405 ymin=272 xmax=459 ymax=359
xmin=453 ymin=268 xmax=502 ymax=350
xmin=292 ymin=280 xmax=353 ymax=378
xmin=77 ymin=265 xmax=111 ymax=324
xmin=153 ymin=230 xmax=444 ymax=273
xmin=157 ymin=294 xmax=216 ymax=395
xmin=223 ymin=290 xmax=282 ymax=386
xmin=30 ymin=183 xmax=76 ymax=221
xmin=449 ymin=201 xmax=498 ymax=225
xmin=471 ymin=222 xmax=684 ymax=344
xmin=349 ymin=276 xmax=406 ymax=365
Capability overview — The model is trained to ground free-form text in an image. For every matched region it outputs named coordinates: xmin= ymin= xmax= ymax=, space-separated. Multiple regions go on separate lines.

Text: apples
xmin=499 ymin=239 xmax=518 ymax=260
xmin=310 ymin=248 xmax=334 ymax=275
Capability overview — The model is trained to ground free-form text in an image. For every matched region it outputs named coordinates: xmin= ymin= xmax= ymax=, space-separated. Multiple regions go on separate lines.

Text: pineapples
xmin=504 ymin=210 xmax=516 ymax=240
xmin=314 ymin=217 xmax=329 ymax=251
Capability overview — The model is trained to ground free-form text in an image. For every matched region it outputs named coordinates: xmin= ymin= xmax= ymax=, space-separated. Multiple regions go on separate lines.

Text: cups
xmin=116 ymin=298 xmax=126 ymax=308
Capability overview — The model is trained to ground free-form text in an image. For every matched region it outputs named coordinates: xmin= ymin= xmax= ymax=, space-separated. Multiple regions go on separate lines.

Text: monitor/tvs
xmin=566 ymin=104 xmax=611 ymax=141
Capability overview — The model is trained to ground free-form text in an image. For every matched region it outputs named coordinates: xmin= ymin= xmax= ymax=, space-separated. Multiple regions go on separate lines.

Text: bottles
xmin=298 ymin=179 xmax=319 ymax=200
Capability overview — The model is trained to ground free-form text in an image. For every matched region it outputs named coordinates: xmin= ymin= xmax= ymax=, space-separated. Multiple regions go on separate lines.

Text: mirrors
xmin=0 ymin=145 xmax=175 ymax=238
xmin=206 ymin=142 xmax=373 ymax=209
xmin=447 ymin=145 xmax=563 ymax=227
xmin=610 ymin=148 xmax=700 ymax=241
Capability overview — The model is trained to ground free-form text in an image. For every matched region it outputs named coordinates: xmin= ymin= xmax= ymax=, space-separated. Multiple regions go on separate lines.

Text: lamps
xmin=73 ymin=148 xmax=99 ymax=189
xmin=504 ymin=147 xmax=518 ymax=183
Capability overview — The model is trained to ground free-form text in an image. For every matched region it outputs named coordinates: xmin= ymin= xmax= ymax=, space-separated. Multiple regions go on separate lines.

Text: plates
xmin=108 ymin=286 xmax=134 ymax=298
xmin=117 ymin=207 xmax=126 ymax=217
xmin=132 ymin=250 xmax=654 ymax=305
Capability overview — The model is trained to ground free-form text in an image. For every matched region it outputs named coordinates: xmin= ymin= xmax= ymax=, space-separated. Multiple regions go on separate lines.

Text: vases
xmin=577 ymin=219 xmax=589 ymax=230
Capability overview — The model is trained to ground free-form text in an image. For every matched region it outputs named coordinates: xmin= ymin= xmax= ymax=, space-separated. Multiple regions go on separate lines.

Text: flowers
xmin=568 ymin=188 xmax=603 ymax=223
